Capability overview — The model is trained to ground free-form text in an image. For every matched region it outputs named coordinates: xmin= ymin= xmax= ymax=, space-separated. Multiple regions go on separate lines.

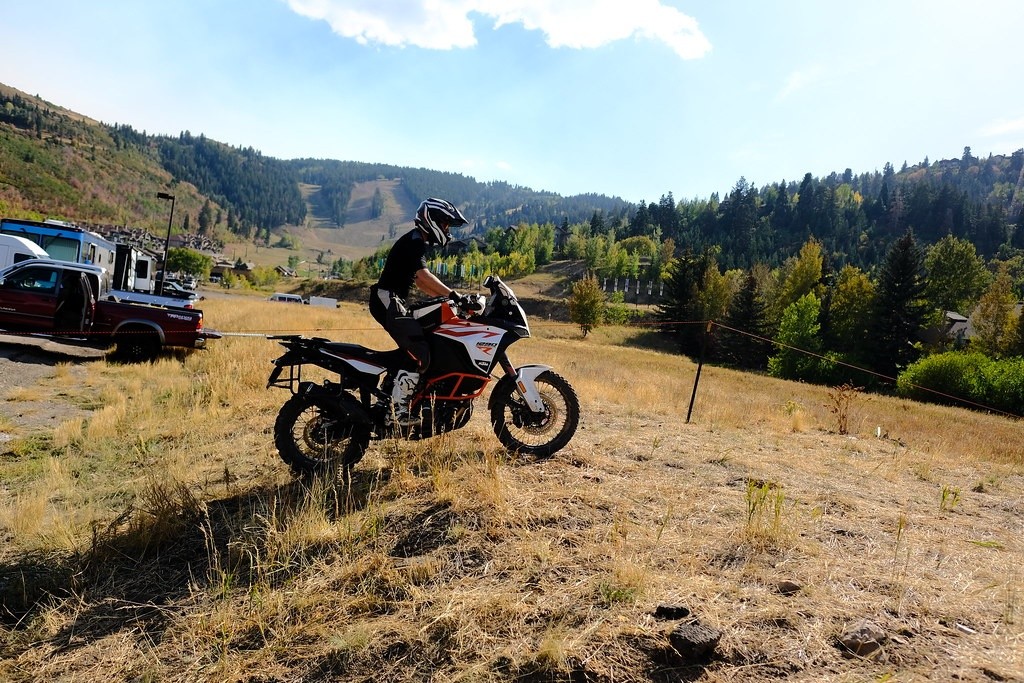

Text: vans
xmin=270 ymin=293 xmax=303 ymax=305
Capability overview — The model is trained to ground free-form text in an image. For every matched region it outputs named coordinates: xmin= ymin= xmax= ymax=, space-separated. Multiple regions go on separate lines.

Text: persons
xmin=370 ymin=198 xmax=482 ymax=427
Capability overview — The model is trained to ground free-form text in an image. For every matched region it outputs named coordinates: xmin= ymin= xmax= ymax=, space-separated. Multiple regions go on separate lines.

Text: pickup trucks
xmin=0 ymin=234 xmax=224 ymax=361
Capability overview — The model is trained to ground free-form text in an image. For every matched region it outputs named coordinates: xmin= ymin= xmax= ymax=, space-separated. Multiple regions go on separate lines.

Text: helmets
xmin=413 ymin=197 xmax=469 ymax=248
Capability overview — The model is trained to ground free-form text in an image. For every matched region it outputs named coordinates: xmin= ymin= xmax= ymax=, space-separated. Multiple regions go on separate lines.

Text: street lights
xmin=156 ymin=191 xmax=176 ymax=298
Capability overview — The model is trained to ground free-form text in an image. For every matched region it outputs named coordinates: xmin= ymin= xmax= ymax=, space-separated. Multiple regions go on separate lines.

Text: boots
xmin=385 ymin=369 xmax=422 ymax=426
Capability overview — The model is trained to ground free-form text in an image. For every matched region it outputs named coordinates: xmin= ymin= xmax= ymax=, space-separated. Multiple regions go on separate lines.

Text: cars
xmin=155 ymin=279 xmax=199 ymax=302
xmin=183 ymin=280 xmax=197 ymax=291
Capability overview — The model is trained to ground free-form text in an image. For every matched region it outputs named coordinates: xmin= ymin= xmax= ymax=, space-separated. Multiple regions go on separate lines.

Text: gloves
xmin=448 ymin=290 xmax=478 ymax=311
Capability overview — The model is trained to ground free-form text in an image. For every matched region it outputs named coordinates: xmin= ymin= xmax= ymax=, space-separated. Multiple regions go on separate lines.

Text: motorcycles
xmin=257 ymin=272 xmax=581 ymax=483
